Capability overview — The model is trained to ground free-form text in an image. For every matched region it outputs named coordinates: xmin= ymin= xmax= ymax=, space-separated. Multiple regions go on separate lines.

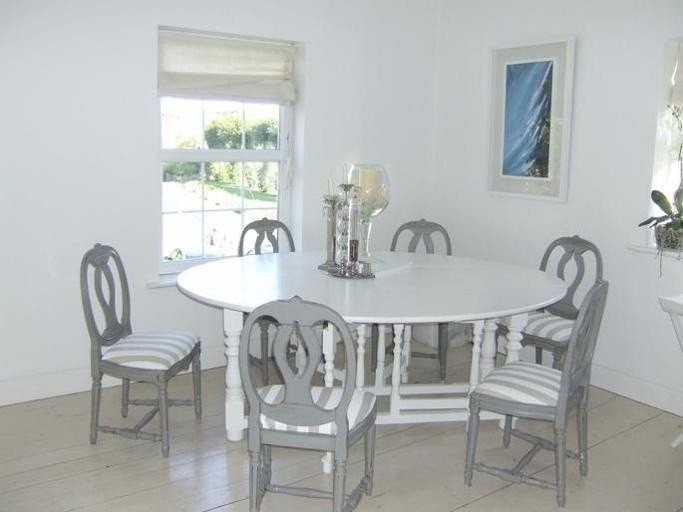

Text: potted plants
xmin=638 ymin=187 xmax=683 ymax=251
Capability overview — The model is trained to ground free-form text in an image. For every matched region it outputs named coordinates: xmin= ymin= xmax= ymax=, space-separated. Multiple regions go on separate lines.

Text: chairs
xmin=79 ymin=244 xmax=203 ymax=456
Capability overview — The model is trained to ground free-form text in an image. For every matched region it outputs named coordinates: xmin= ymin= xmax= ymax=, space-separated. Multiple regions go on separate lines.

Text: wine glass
xmin=349 ymin=163 xmax=390 ymax=266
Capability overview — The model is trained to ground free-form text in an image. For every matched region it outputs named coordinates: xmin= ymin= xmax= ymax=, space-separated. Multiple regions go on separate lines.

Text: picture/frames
xmin=486 ymin=35 xmax=576 ymax=204
